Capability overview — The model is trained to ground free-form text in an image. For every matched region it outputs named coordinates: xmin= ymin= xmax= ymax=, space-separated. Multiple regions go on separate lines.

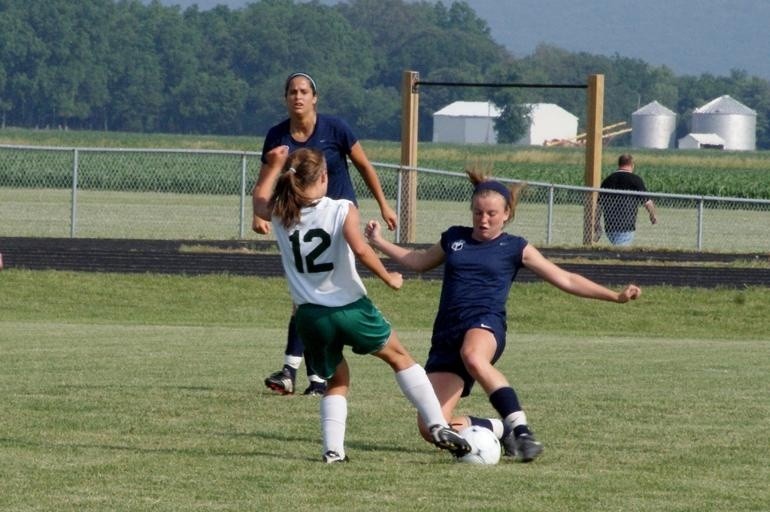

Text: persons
xmin=593 ymin=153 xmax=658 ymax=247
xmin=252 ymin=70 xmax=399 ymax=395
xmin=362 ymin=168 xmax=644 ymax=464
xmin=252 ymin=144 xmax=472 ymax=466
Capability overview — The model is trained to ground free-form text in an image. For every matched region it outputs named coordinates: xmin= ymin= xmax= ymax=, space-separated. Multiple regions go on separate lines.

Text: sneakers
xmin=264 ymin=367 xmax=295 ymax=394
xmin=322 ymin=451 xmax=349 ymax=465
xmin=502 ymin=419 xmax=518 ymax=460
xmin=304 ymin=381 xmax=326 ymax=395
xmin=518 ymin=434 xmax=542 ymax=462
xmin=428 ymin=424 xmax=471 ymax=459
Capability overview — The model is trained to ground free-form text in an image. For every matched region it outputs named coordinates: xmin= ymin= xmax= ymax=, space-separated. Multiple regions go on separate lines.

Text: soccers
xmin=452 ymin=425 xmax=502 ymax=463
